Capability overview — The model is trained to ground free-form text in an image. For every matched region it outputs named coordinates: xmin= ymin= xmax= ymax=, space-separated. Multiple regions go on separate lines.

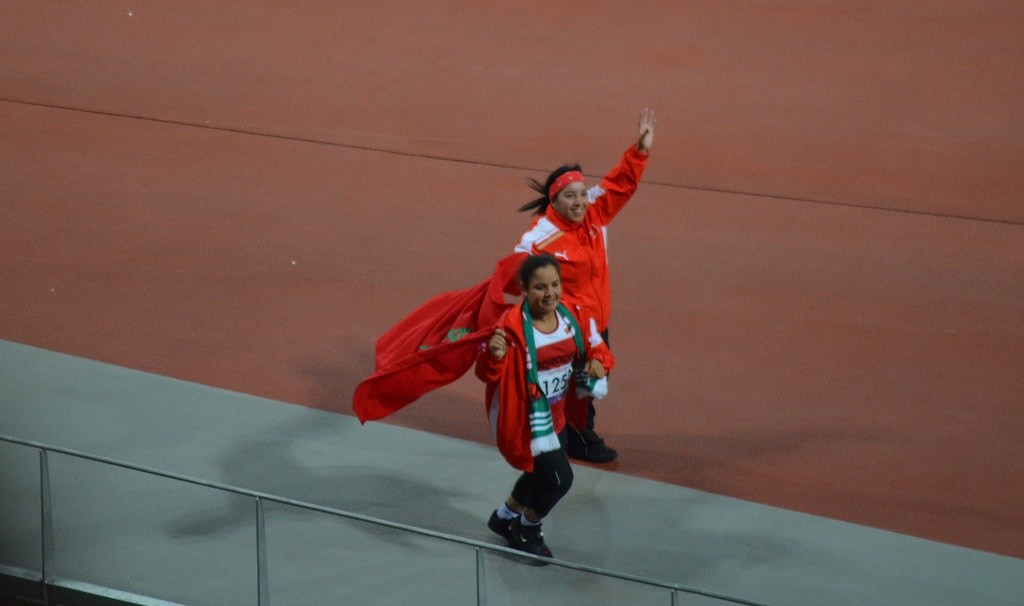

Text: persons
xmin=475 ymin=255 xmax=605 ymax=564
xmin=515 ymin=107 xmax=654 ymax=463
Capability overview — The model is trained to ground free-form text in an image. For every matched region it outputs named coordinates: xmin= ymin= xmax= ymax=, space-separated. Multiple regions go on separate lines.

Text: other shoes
xmin=568 ymin=442 xmax=618 ymax=464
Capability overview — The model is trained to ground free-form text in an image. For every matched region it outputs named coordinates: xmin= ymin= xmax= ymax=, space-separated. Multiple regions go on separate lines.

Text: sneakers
xmin=487 ymin=510 xmax=510 ymax=543
xmin=507 ymin=516 xmax=553 ymax=565
